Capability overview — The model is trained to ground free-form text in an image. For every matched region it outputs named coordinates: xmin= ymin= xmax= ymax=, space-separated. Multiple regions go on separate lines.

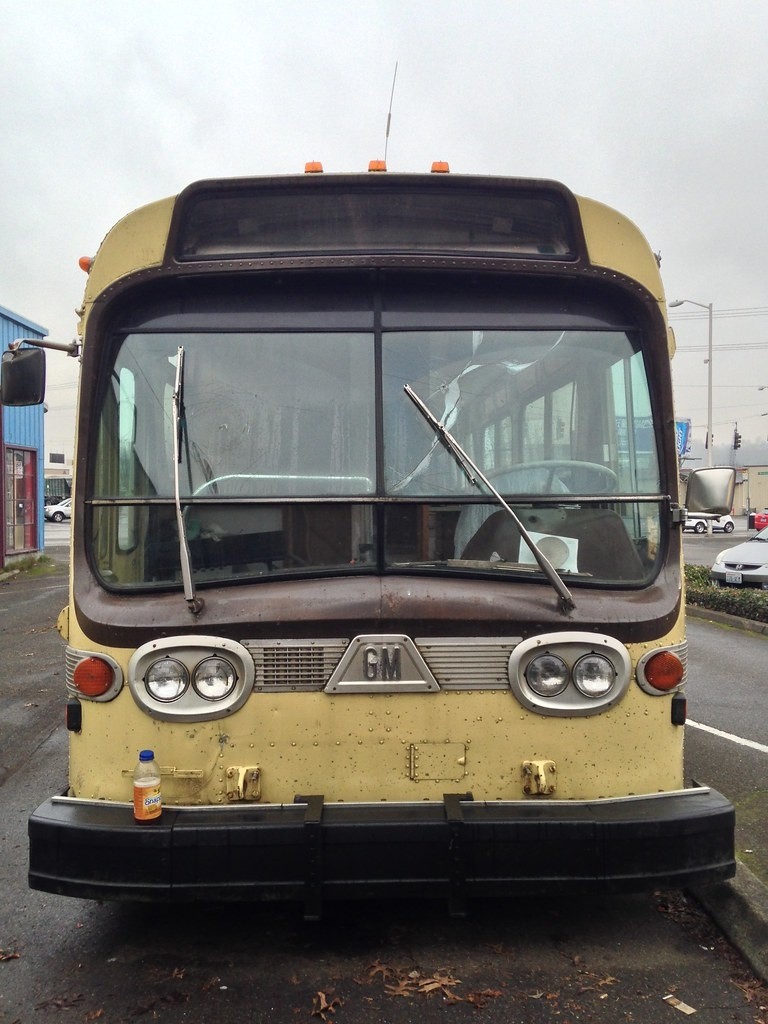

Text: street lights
xmin=666 ymin=299 xmax=714 ymax=470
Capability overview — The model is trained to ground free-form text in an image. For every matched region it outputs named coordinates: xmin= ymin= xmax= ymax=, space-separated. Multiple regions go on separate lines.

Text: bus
xmin=0 ymin=160 xmax=740 ymax=907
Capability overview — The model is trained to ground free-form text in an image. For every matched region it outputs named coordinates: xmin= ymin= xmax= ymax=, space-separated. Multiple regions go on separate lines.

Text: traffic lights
xmin=734 ymin=433 xmax=741 ymax=450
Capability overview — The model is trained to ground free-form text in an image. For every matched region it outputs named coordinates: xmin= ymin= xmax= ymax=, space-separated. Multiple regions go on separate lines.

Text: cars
xmin=44 ymin=497 xmax=72 ymax=523
xmin=755 ymin=508 xmax=768 ymax=530
xmin=683 ymin=505 xmax=734 ymax=534
xmin=710 ymin=523 xmax=768 ymax=593
